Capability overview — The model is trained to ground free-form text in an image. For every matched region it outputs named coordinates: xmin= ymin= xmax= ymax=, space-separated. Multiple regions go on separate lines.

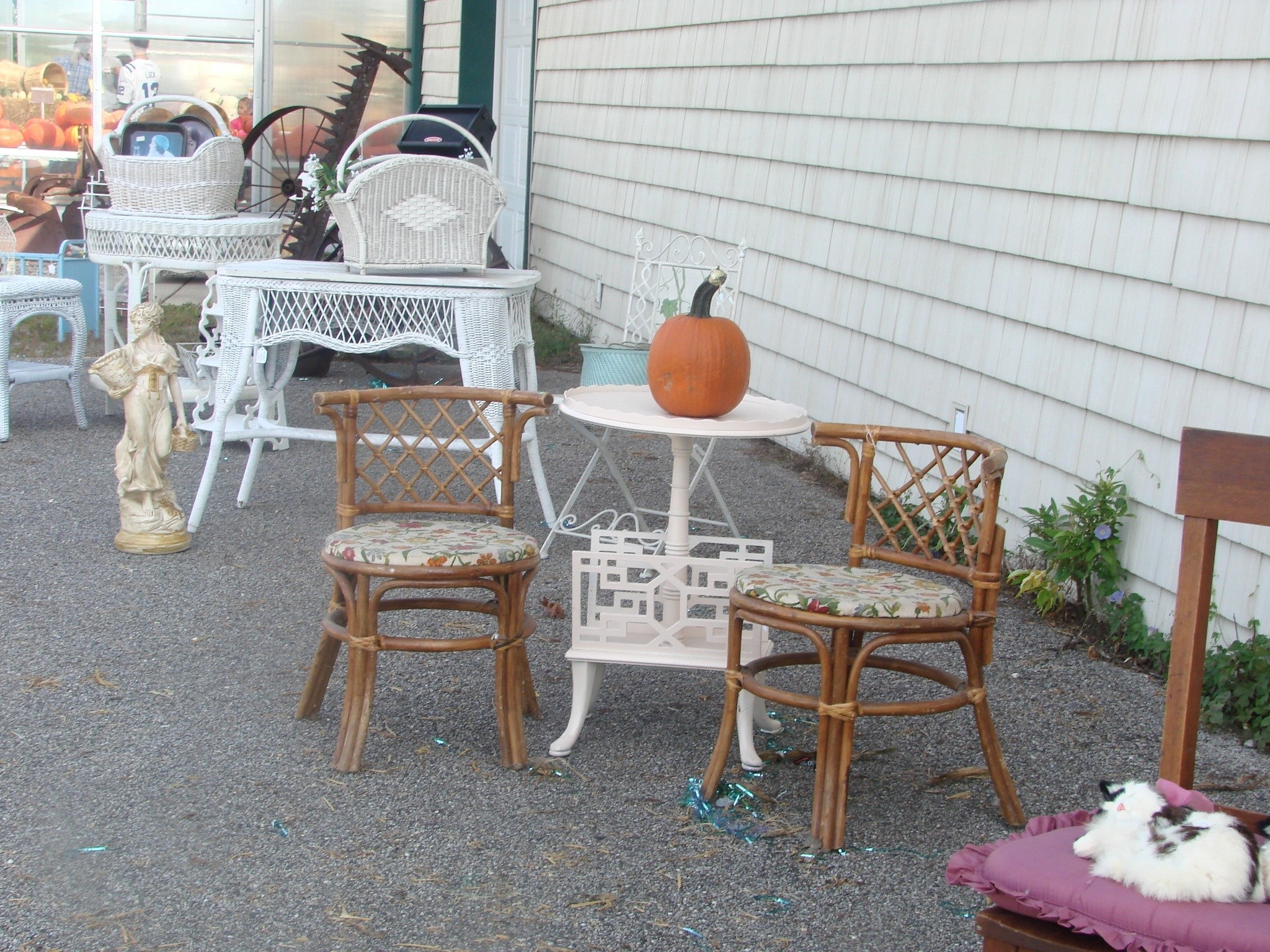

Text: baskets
xmin=102 ymin=95 xmax=244 ymax=219
xmin=324 ymin=114 xmax=507 ymax=278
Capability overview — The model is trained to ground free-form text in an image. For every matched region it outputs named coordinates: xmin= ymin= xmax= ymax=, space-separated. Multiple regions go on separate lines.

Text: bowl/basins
xmin=119 ymin=121 xmax=186 ymax=158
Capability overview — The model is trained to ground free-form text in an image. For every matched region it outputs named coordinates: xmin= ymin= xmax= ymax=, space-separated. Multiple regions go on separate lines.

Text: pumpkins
xmin=648 ymin=268 xmax=750 ymax=419
xmin=0 ymin=100 xmax=403 ymax=188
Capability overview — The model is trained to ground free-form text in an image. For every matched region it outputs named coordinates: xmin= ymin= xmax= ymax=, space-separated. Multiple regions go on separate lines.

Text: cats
xmin=1071 ymin=780 xmax=1269 ymax=905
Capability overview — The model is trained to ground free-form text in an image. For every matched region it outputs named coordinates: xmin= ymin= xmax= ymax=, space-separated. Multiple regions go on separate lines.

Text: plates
xmin=165 ymin=115 xmax=217 ymax=157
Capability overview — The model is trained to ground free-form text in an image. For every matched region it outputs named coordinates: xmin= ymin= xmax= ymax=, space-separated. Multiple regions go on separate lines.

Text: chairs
xmin=539 ymin=224 xmax=755 ymax=560
xmin=0 ymin=213 xmax=88 ymax=442
xmin=699 ymin=422 xmax=1026 ymax=854
xmin=945 ymin=427 xmax=1270 ymax=952
xmin=295 ymin=385 xmax=555 ymax=773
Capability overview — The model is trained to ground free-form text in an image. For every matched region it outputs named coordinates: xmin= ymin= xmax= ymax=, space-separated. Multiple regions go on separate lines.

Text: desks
xmin=549 ymin=384 xmax=809 ymax=772
xmin=84 ymin=209 xmax=283 ymax=417
xmin=186 ymin=258 xmax=556 ymax=531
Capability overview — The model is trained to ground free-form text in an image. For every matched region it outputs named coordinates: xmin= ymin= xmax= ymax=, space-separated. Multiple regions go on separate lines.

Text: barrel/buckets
xmin=0 ymin=60 xmax=26 ymax=93
xmin=24 ymin=63 xmax=68 ymax=97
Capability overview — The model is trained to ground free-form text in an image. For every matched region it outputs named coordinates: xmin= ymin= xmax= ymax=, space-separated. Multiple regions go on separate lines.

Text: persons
xmin=183 ymin=129 xmax=200 ymax=158
xmin=106 ymin=29 xmax=161 ymax=120
xmin=229 ymin=97 xmax=256 ymax=152
xmin=88 ymin=297 xmax=189 ymax=534
xmin=53 ymin=34 xmax=96 ymax=92
xmin=110 ymin=52 xmax=131 ymax=93
xmin=89 ymin=34 xmax=124 ymax=97
xmin=148 ymin=135 xmax=174 ymax=158
